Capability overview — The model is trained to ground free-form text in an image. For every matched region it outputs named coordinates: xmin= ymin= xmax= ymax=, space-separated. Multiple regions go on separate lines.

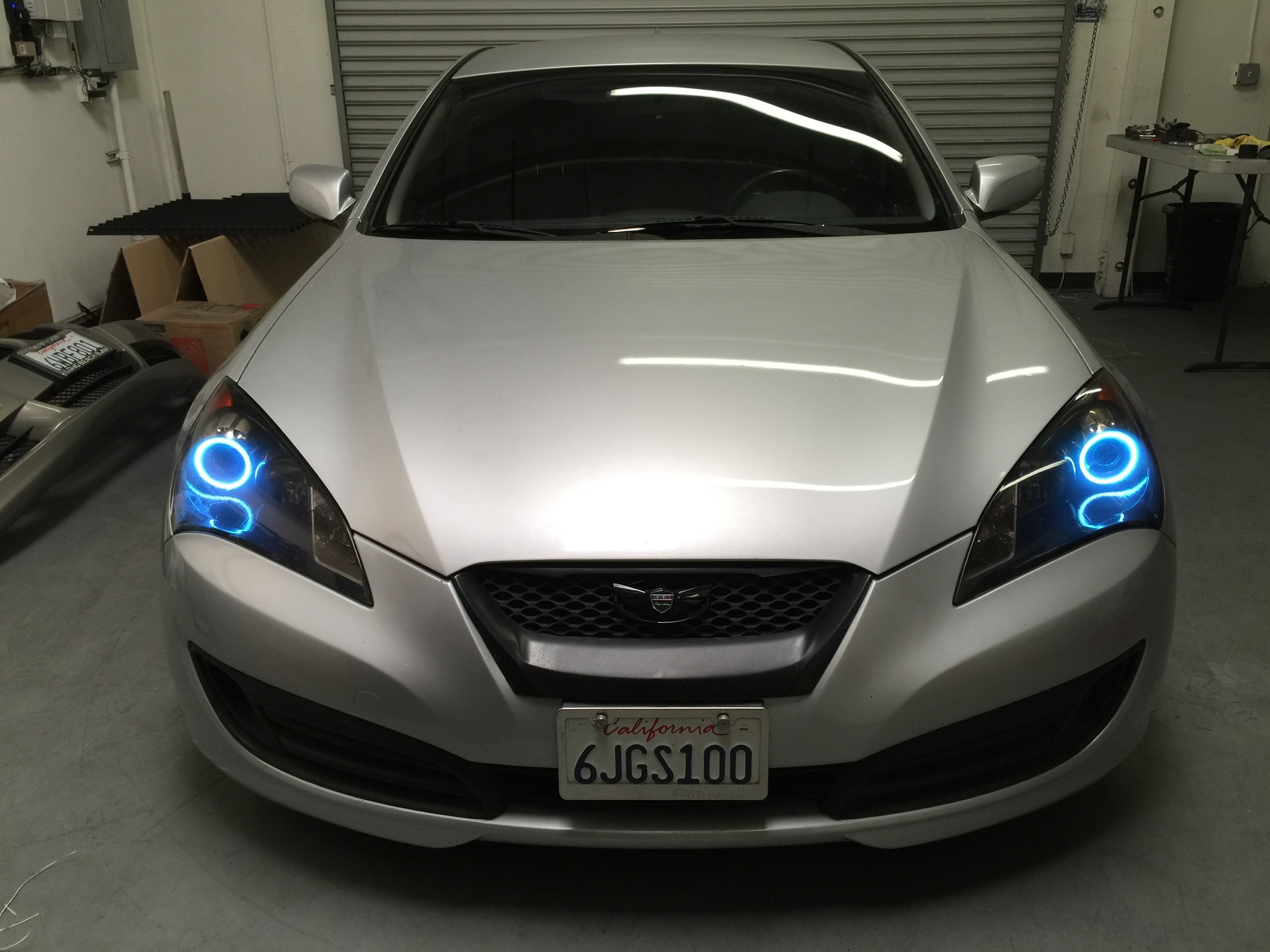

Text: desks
xmin=1094 ymin=134 xmax=1270 ymax=374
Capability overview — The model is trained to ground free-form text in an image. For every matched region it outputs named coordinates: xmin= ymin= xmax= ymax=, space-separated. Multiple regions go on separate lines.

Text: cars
xmin=160 ymin=28 xmax=1181 ymax=851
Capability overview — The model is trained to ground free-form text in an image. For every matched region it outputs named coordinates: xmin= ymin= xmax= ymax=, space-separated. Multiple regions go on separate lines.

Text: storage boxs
xmin=134 ymin=301 xmax=271 ymax=380
xmin=0 ymin=277 xmax=55 ymax=339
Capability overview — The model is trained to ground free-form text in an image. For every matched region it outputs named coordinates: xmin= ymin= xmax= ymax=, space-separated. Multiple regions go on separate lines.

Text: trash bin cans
xmin=1161 ymin=201 xmax=1251 ymax=306
